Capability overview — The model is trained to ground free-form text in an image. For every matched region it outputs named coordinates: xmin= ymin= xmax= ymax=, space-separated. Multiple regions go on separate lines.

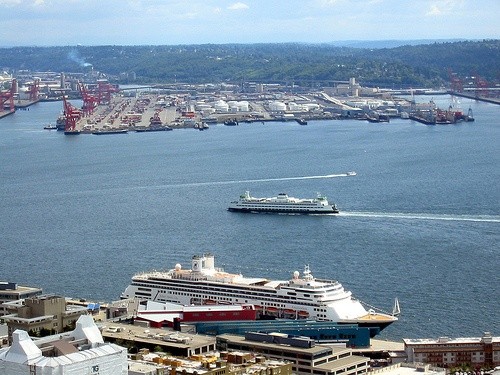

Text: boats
xmin=227 ymin=189 xmax=340 ymax=213
xmin=134 ymin=128 xmax=173 ymax=132
xmin=93 ymin=129 xmax=128 ymax=134
xmin=347 ymin=172 xmax=356 ymax=176
xmin=120 ymin=253 xmax=400 ymax=338
xmin=44 ymin=124 xmax=57 ymax=129
xmin=224 ymin=118 xmax=239 ymax=125
xmin=55 ymin=116 xmax=67 ymax=130
